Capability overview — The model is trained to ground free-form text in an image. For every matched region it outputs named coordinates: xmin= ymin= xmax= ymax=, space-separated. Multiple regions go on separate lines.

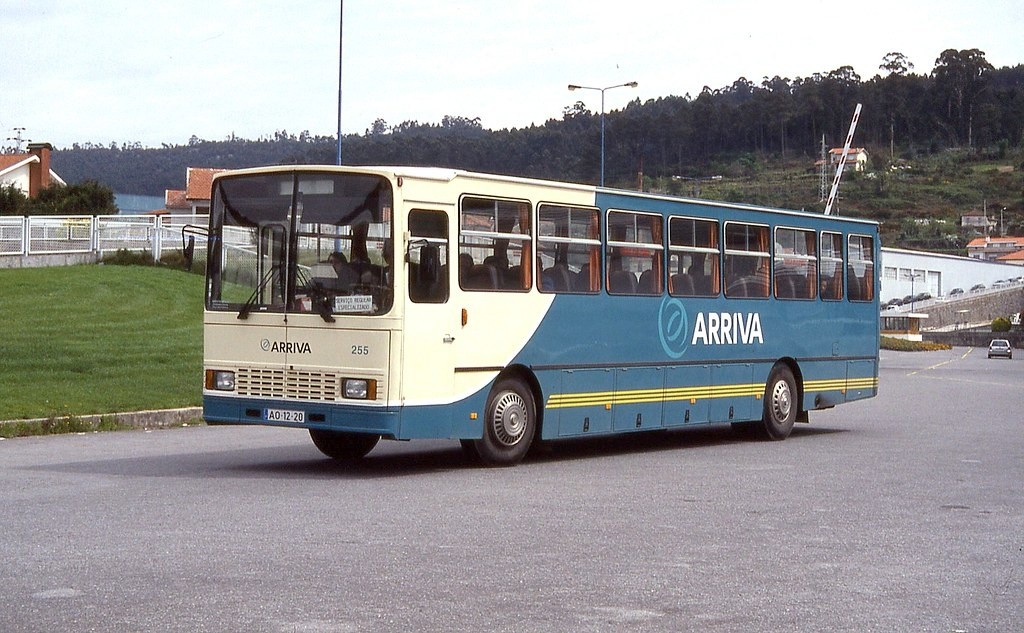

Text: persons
xmin=820 ymin=275 xmax=835 ymax=297
xmin=307 ymin=237 xmax=552 ymax=313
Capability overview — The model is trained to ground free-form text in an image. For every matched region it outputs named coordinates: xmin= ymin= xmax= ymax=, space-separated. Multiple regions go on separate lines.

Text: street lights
xmin=567 ymin=80 xmax=638 ymax=189
xmin=1001 ymin=206 xmax=1007 ymax=239
xmin=903 ymin=273 xmax=921 ymax=313
xmin=671 ymin=174 xmax=722 ymax=198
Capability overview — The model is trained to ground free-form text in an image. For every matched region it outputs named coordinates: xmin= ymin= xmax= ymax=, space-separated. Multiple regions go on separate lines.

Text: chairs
xmin=317 ymin=245 xmax=845 ymax=299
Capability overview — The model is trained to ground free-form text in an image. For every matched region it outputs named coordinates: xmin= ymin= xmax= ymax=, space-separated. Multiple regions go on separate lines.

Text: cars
xmin=969 ymin=284 xmax=986 ymax=291
xmin=949 ymin=287 xmax=964 ymax=294
xmin=992 ymin=276 xmax=1024 ymax=287
xmin=889 ymin=297 xmax=904 ymax=307
xmin=880 ymin=300 xmax=888 ymax=310
xmin=987 ymin=339 xmax=1013 ymax=360
xmin=902 ymin=295 xmax=919 ymax=305
xmin=916 ymin=292 xmax=932 ymax=302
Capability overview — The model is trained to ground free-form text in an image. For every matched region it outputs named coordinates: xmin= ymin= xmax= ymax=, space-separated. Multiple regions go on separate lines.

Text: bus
xmin=181 ymin=166 xmax=881 ymax=470
